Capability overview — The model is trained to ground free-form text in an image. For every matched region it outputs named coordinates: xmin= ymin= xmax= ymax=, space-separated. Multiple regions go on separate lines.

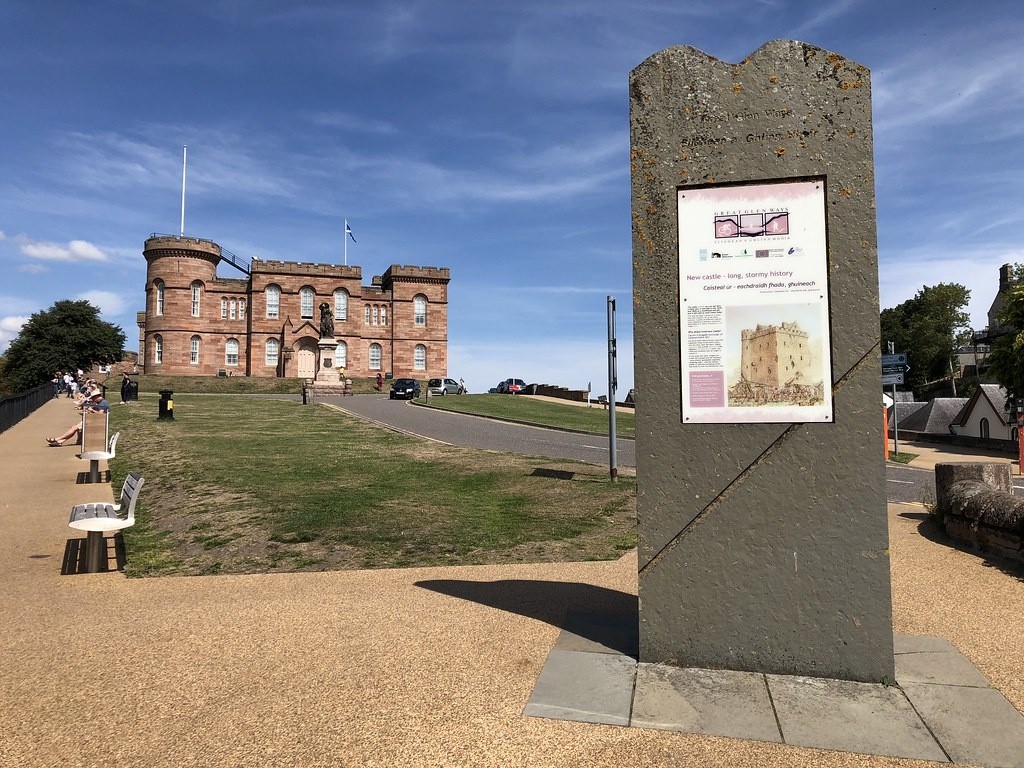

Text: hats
xmin=91 ymin=384 xmax=96 ymax=386
xmin=88 ymin=390 xmax=101 ymax=398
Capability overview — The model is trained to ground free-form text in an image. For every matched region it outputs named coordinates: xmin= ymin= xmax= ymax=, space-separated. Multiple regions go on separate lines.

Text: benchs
xmin=75 ymin=432 xmax=121 ymax=482
xmin=67 ymin=472 xmax=145 ymax=572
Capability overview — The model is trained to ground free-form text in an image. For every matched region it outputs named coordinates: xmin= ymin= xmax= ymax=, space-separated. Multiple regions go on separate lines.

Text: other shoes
xmin=125 ymin=401 xmax=129 ymax=404
xmin=120 ymin=402 xmax=124 ymax=404
xmin=71 ymin=395 xmax=74 ymax=399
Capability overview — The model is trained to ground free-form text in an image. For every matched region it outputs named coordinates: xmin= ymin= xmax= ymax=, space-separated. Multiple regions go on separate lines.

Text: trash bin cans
xmin=158 ymin=389 xmax=175 ymax=420
xmin=82 ymin=403 xmax=108 ymax=452
xmin=385 ymin=372 xmax=393 ymax=379
xmin=219 ymin=369 xmax=226 ymax=376
xmin=127 ymin=381 xmax=138 ymax=400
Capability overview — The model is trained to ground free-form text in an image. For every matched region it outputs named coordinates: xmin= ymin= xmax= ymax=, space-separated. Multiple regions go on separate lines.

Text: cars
xmin=488 ymin=387 xmax=498 ymax=393
xmin=502 ymin=378 xmax=526 ymax=394
xmin=428 ymin=377 xmax=463 ymax=396
xmin=389 ymin=378 xmax=420 ymax=400
xmin=496 ymin=381 xmax=506 ymax=393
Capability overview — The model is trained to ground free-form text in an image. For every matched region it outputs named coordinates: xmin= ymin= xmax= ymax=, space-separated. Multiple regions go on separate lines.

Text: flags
xmin=346 ymin=224 xmax=357 ymax=242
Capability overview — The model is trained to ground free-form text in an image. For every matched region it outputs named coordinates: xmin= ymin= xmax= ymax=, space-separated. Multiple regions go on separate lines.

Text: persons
xmin=120 ymin=372 xmax=130 ymax=404
xmin=320 ymin=304 xmax=334 ymax=339
xmin=74 ymin=378 xmax=100 ymax=414
xmin=46 ymin=390 xmax=109 ymax=447
xmin=377 ymin=373 xmax=383 ymax=390
xmin=106 ymin=363 xmax=111 ymax=378
xmin=53 ymin=368 xmax=83 ymax=398
xmin=340 ymin=366 xmax=344 ymax=378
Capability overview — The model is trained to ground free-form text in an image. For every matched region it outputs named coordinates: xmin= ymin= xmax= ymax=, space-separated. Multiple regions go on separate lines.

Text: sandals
xmin=46 ymin=438 xmax=57 ymax=443
xmin=48 ymin=442 xmax=62 ymax=447
